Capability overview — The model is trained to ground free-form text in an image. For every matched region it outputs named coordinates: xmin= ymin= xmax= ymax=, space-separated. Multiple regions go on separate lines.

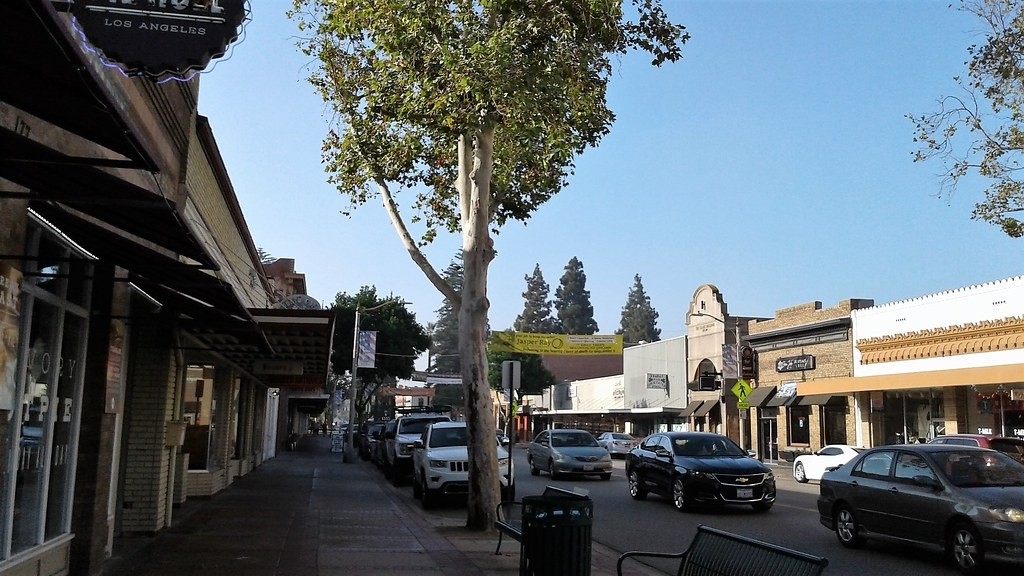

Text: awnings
xmin=677 ymin=399 xmax=720 ymax=417
xmin=742 ymin=386 xmax=846 ymax=407
xmin=0 ymin=0 xmax=339 ymax=390
xmin=796 ymin=363 xmax=1024 ymax=398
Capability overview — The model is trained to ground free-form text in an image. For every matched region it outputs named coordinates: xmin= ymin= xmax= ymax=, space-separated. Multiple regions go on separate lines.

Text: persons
xmin=910 ymin=432 xmax=920 ymax=443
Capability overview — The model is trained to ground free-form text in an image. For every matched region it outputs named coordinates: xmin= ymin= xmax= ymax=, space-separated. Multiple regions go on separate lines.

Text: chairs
xmin=431 ymin=432 xmax=448 ymax=446
xmin=553 ymin=438 xmax=560 ymax=445
xmin=566 ymin=434 xmax=576 ymax=444
xmin=866 ymin=459 xmax=887 ymax=474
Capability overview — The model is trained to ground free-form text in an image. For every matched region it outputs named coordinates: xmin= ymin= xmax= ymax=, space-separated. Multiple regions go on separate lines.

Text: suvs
xmin=340 ymin=404 xmax=514 ymax=513
xmin=898 ymin=434 xmax=1024 ymax=483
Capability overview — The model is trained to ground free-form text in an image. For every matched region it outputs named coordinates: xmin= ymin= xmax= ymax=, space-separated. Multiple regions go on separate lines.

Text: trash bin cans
xmin=519 ymin=495 xmax=594 ymax=576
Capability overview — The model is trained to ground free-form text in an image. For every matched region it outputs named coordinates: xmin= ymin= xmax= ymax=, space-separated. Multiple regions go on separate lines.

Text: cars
xmin=625 ymin=431 xmax=776 ymax=514
xmin=793 ymin=445 xmax=891 ymax=483
xmin=591 ymin=432 xmax=638 ymax=459
xmin=526 ymin=430 xmax=613 ymax=481
xmin=816 ymin=444 xmax=1024 ymax=575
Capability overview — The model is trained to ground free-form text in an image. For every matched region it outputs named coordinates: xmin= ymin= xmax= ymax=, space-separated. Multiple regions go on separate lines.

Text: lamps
xmin=932 ymin=390 xmax=936 ymax=398
xmin=895 ymin=391 xmax=900 ymax=398
xmin=885 ymin=392 xmax=891 ymax=398
xmin=907 ymin=391 xmax=914 ymax=398
xmin=919 ymin=390 xmax=926 ymax=397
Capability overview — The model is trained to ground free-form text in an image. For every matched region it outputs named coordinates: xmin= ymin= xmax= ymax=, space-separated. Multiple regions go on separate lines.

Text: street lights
xmin=689 ymin=313 xmax=745 ymax=450
xmin=343 ymin=300 xmax=413 ymax=463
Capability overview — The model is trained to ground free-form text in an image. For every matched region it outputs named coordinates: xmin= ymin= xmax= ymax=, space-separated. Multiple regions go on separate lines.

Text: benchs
xmin=616 ymin=523 xmax=829 ymax=576
xmin=493 ymin=486 xmax=589 ymax=576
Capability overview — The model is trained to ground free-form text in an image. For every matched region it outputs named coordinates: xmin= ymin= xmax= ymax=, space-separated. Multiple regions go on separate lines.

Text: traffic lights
xmin=699 ymin=374 xmax=715 ymax=392
xmin=742 ymin=345 xmax=755 ymax=380
